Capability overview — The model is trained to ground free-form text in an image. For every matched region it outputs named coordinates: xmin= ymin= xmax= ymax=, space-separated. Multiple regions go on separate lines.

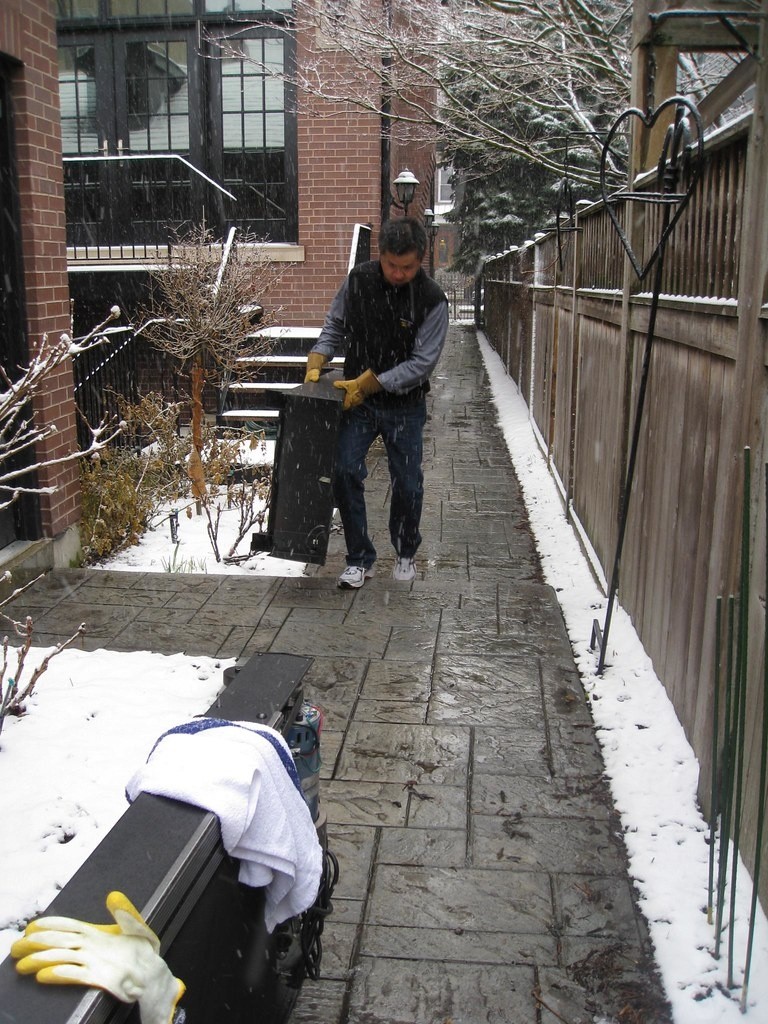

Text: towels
xmin=124 ymin=716 xmax=324 ymax=935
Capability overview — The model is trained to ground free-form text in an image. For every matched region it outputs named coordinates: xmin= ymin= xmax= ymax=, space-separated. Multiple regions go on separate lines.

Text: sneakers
xmin=392 ymin=553 xmax=416 ymax=584
xmin=337 ymin=565 xmax=375 ymax=589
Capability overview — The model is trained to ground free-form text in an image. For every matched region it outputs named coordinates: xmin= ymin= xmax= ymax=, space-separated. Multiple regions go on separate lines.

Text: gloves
xmin=333 ymin=368 xmax=382 ymax=410
xmin=10 ymin=891 xmax=186 ymax=1024
xmin=303 ymin=352 xmax=329 ymax=384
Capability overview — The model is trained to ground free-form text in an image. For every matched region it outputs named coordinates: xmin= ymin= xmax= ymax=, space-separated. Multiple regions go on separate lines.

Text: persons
xmin=305 ymin=219 xmax=449 ymax=592
